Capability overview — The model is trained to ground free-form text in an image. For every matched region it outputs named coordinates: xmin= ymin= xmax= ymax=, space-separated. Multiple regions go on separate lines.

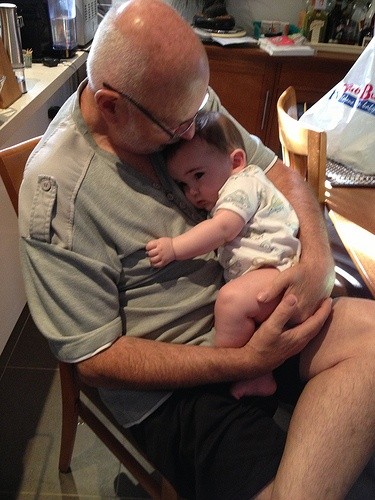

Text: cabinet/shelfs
xmin=201 ymin=42 xmax=361 ymax=161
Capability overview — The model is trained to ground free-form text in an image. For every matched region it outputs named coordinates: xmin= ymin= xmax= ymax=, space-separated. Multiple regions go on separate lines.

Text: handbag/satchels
xmin=298 ymin=35 xmax=375 ymax=175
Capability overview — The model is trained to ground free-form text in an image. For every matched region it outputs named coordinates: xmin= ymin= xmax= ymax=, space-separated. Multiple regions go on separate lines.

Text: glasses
xmin=103 ymin=82 xmax=210 ymax=141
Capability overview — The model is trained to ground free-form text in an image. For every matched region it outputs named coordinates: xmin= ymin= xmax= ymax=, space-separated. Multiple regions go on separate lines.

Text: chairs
xmin=0 ymin=133 xmax=185 ymax=500
xmin=276 ymin=84 xmax=330 ymax=201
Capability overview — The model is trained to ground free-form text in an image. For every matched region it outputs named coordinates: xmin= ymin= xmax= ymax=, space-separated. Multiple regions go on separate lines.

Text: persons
xmin=18 ymin=0 xmax=375 ymax=500
xmin=145 ymin=110 xmax=301 ymax=399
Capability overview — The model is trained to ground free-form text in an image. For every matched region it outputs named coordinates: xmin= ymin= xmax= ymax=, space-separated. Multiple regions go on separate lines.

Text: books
xmin=258 ymin=38 xmax=313 ymax=56
